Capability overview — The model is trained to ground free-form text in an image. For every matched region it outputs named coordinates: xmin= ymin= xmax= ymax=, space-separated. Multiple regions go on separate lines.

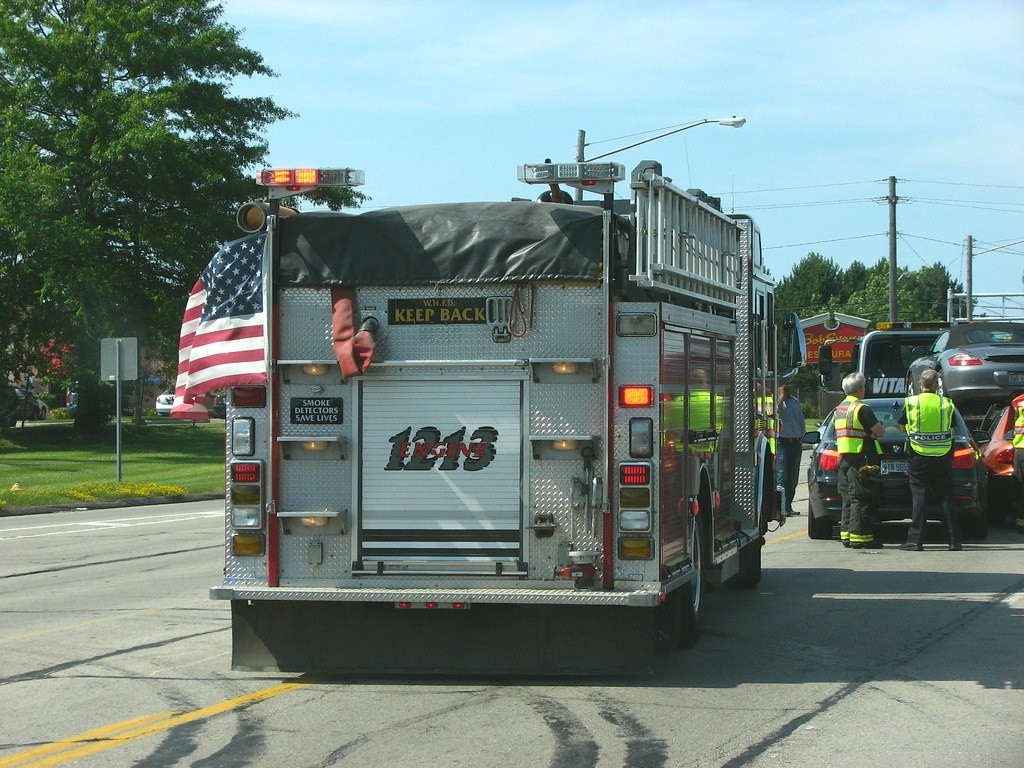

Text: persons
xmin=776 ymin=383 xmax=805 ymax=516
xmin=661 ymin=368 xmax=777 ymax=462
xmin=831 ymin=373 xmax=884 ymax=549
xmin=1004 ymin=392 xmax=1024 ymax=532
xmin=898 ymin=370 xmax=962 ymax=552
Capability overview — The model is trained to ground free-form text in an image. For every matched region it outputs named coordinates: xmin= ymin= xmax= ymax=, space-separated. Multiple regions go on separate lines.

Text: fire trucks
xmin=167 ymin=156 xmax=808 ymax=659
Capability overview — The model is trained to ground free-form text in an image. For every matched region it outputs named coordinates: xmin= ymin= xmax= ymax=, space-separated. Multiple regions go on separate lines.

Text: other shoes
xmin=783 ymin=510 xmax=800 ymax=517
xmin=845 ymin=541 xmax=882 ymax=549
xmin=949 ymin=544 xmax=962 ymax=551
xmin=899 ymin=542 xmax=923 ymax=551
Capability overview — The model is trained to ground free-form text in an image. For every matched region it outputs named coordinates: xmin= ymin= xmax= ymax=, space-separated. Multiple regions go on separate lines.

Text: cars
xmin=213 ymin=390 xmax=227 ymax=419
xmin=812 ymin=406 xmax=839 ymax=449
xmin=66 ymin=381 xmax=79 ymax=412
xmin=0 ymin=382 xmax=48 ymax=429
xmin=971 ymin=392 xmax=1024 ymax=528
xmin=802 ymin=394 xmax=992 ymax=541
xmin=906 ymin=320 xmax=1024 ymax=415
xmin=155 ymin=388 xmax=175 ymax=417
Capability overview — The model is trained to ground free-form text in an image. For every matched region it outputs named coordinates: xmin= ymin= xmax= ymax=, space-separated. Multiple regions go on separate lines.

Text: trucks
xmin=820 ymin=319 xmax=953 ymax=400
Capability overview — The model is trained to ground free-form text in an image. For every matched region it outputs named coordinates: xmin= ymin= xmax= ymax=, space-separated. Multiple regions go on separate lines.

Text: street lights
xmin=571 ymin=113 xmax=748 ymax=206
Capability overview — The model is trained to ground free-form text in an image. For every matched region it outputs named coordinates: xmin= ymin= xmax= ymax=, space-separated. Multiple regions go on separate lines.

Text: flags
xmin=170 ymin=232 xmax=271 ymax=421
